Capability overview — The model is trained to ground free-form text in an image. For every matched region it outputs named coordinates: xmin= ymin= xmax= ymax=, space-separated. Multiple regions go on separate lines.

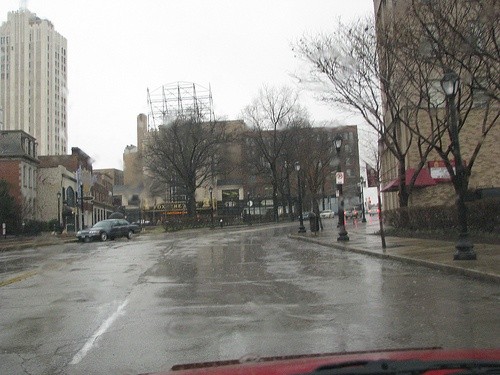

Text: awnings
xmin=380 ymin=166 xmax=435 ymax=195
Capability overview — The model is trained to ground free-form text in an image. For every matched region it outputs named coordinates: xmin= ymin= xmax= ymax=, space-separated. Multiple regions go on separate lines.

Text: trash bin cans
xmin=309 ymin=215 xmax=319 ymax=232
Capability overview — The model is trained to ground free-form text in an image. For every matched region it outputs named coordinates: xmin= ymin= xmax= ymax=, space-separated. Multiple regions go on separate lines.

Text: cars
xmin=76 ymin=219 xmax=140 ymax=243
xmin=320 ymin=210 xmax=334 ymax=219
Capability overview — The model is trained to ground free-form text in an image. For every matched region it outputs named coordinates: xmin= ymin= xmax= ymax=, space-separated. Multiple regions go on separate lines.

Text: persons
xmin=219 ymin=218 xmax=223 ymax=228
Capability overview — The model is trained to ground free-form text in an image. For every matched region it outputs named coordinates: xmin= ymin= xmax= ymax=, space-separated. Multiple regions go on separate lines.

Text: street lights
xmin=293 ymin=161 xmax=306 ymax=233
xmin=440 ymin=66 xmax=477 ymax=260
xmin=332 ymin=132 xmax=349 ymax=242
xmin=247 ymin=191 xmax=252 ymax=226
xmin=209 ymin=186 xmax=215 ymax=230
xmin=360 ymin=175 xmax=366 ymax=222
xmin=56 ymin=190 xmax=62 ymax=238
